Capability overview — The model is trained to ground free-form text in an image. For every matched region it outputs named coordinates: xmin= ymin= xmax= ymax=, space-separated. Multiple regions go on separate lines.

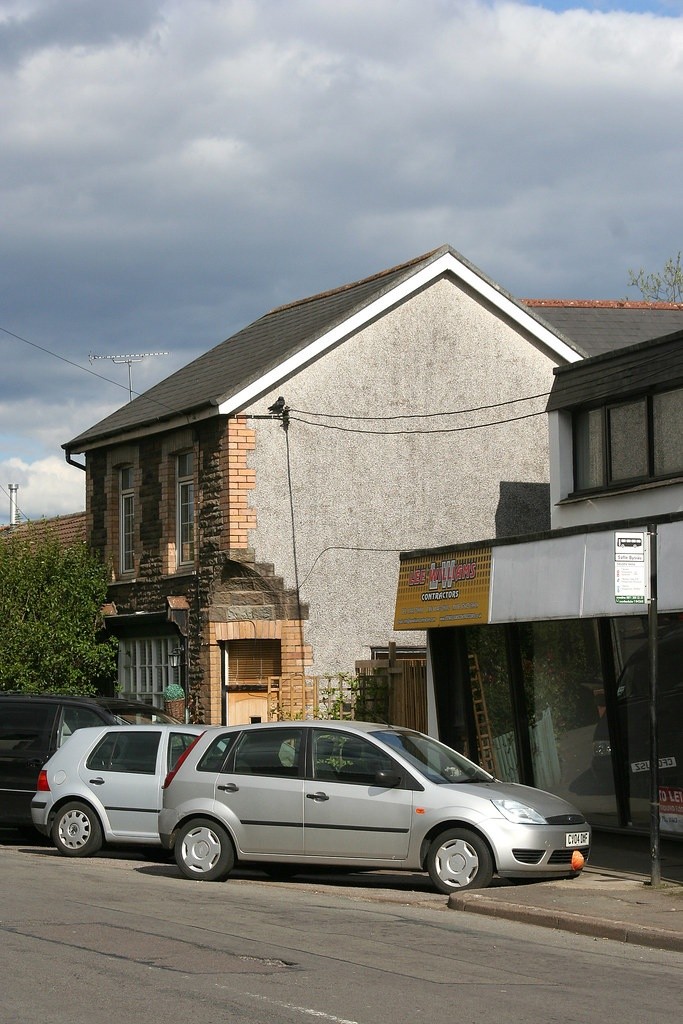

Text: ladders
xmin=469 ymin=654 xmax=498 ymax=778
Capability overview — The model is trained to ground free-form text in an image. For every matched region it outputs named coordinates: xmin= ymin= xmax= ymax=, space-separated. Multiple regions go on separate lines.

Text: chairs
xmin=339 ymin=740 xmax=373 ymax=782
xmin=317 ymin=737 xmax=338 ymax=779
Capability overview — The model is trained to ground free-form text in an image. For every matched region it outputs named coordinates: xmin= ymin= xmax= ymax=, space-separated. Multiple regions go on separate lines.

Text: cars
xmin=157 ymin=720 xmax=591 ymax=896
xmin=592 ymin=628 xmax=683 ymax=795
xmin=31 ymin=724 xmax=296 ymax=858
xmin=0 ymin=692 xmax=213 ymax=844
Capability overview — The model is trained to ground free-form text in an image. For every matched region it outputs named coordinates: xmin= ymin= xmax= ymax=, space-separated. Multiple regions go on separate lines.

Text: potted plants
xmin=163 ymin=683 xmax=185 ymax=722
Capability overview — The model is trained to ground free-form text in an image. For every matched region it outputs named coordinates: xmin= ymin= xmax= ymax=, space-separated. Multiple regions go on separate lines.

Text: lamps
xmin=168 ymin=647 xmax=184 ymax=669
